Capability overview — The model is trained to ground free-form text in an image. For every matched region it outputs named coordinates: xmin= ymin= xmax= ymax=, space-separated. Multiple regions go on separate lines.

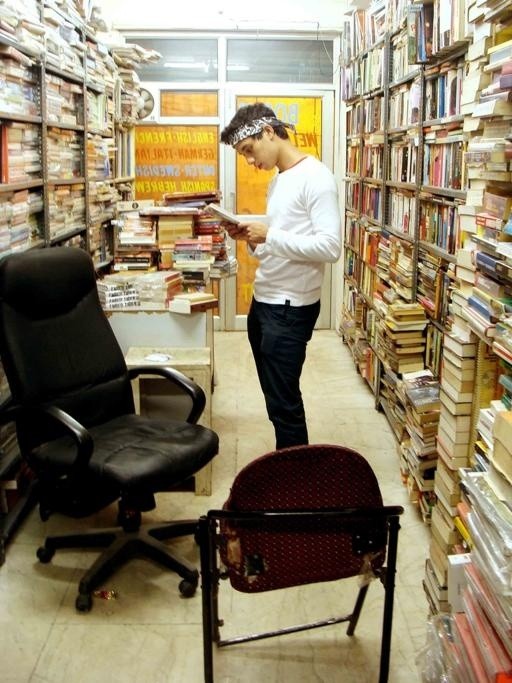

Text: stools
xmin=125 ymin=346 xmax=215 ymax=497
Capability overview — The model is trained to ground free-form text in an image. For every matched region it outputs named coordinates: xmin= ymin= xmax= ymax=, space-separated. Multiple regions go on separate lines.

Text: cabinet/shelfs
xmin=0 ymin=0 xmax=135 ymax=566
xmin=337 ymin=1 xmax=512 ymax=682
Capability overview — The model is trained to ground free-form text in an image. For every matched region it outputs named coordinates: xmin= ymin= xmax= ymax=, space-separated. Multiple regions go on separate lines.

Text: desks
xmin=97 ymin=278 xmax=218 ymax=394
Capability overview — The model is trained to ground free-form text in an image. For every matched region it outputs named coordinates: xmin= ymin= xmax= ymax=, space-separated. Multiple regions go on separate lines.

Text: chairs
xmin=193 ymin=445 xmax=405 ymax=682
xmin=1 ymin=246 xmax=221 ymax=612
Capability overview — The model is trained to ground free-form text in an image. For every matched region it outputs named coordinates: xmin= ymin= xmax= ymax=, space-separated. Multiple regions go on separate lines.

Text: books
xmin=2 ymin=0 xmax=238 ymax=314
xmin=2 ymin=369 xmax=20 ymax=475
xmin=338 ymin=0 xmax=512 ymax=683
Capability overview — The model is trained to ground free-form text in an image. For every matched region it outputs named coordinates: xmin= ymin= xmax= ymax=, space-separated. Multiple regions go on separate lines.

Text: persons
xmin=220 ymin=101 xmax=343 ymax=452
